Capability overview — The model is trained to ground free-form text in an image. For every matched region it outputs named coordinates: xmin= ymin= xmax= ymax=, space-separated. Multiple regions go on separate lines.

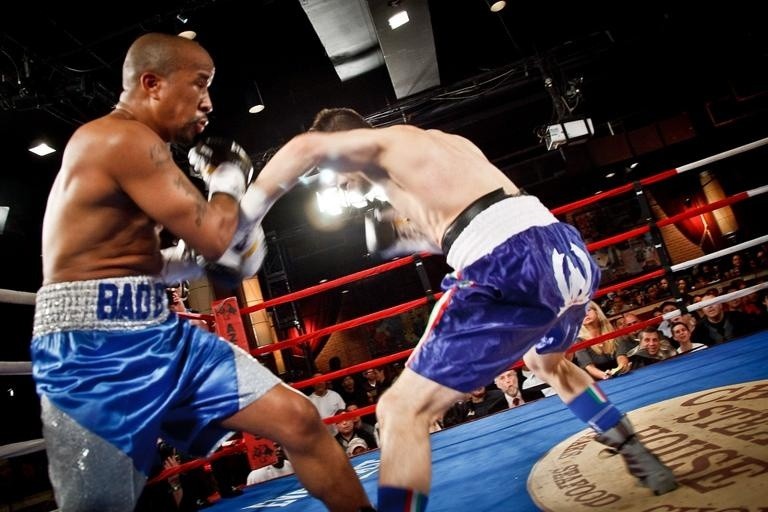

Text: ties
xmin=513 ymin=398 xmax=520 ymax=406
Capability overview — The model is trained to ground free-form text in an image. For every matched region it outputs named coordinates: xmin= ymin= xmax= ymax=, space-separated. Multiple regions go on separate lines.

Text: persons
xmin=27 ymin=30 xmax=376 ymax=512
xmin=134 ymin=243 xmax=768 ymax=512
xmin=227 ymin=107 xmax=682 ymax=512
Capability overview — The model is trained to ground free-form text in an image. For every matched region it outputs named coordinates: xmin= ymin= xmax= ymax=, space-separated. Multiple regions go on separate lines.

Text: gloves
xmin=199 ymin=196 xmax=270 ymax=291
xmin=364 ymin=203 xmax=444 ymax=261
xmin=187 ymin=136 xmax=255 ymax=197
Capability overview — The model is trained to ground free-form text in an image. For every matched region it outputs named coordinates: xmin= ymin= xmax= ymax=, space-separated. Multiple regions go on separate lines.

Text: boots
xmin=594 ymin=411 xmax=678 ymax=495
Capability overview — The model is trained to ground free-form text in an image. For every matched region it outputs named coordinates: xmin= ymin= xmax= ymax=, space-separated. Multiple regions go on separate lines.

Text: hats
xmin=346 ymin=437 xmax=368 ymax=457
